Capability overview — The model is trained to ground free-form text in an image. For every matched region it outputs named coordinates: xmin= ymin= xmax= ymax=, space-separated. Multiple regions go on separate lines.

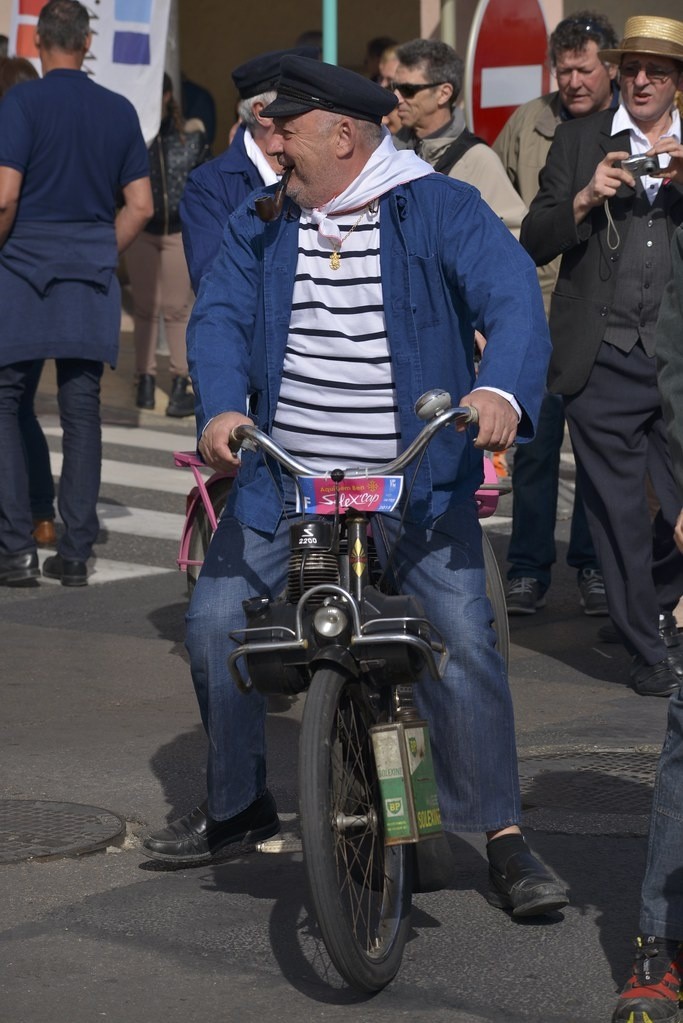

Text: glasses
xmin=619 ymin=62 xmax=677 ymax=79
xmin=390 ymin=80 xmax=446 ymax=98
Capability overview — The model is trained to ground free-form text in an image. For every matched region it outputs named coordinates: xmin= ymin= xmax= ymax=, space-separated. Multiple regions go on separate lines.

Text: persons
xmin=0 ymin=56 xmax=58 ymax=549
xmin=0 ymin=0 xmax=155 ymax=590
xmin=490 ymin=9 xmax=622 ymax=616
xmin=518 ymin=14 xmax=683 ymax=697
xmin=179 ymin=45 xmax=321 ymax=298
xmin=347 ymin=36 xmax=529 ymax=380
xmin=282 ymin=28 xmax=323 ymax=59
xmin=139 ymin=53 xmax=570 ymax=921
xmin=124 ymin=72 xmax=209 ymax=417
xmin=608 ymin=503 xmax=683 ymax=1023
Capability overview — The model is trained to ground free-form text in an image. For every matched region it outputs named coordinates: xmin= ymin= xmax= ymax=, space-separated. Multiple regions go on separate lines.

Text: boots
xmin=137 ymin=374 xmax=156 ymax=409
xmin=166 ymin=375 xmax=195 ymax=416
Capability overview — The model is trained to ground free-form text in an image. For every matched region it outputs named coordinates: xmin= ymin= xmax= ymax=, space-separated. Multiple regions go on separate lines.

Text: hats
xmin=259 ymin=54 xmax=399 ymax=126
xmin=597 ymin=15 xmax=683 ymax=65
xmin=232 ymin=45 xmax=320 ymax=99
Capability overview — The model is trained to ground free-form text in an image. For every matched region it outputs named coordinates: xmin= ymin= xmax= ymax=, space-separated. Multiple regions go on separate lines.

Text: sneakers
xmin=506 ymin=577 xmax=547 ymax=613
xmin=577 ymin=567 xmax=609 ymax=615
xmin=615 ymin=935 xmax=683 ymax=1023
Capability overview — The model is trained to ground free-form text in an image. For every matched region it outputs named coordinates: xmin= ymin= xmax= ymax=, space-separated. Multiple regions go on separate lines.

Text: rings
xmin=211 ymin=451 xmax=218 ymax=458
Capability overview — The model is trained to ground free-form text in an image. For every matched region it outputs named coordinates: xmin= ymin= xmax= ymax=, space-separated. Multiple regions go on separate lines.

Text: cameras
xmin=621 ymin=154 xmax=660 ymax=178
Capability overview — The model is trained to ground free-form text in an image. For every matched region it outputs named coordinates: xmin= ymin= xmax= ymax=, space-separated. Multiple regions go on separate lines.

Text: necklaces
xmin=326 ymin=200 xmax=376 ymax=270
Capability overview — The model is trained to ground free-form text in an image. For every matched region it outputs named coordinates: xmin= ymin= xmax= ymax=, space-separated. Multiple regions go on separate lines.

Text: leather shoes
xmin=140 ymin=788 xmax=281 ymax=863
xmin=658 ymin=610 xmax=683 ymax=679
xmin=628 ymin=653 xmax=681 ymax=696
xmin=488 ymin=851 xmax=570 ymax=916
xmin=0 ymin=551 xmax=40 ymax=583
xmin=43 ymin=554 xmax=88 ymax=586
xmin=32 ymin=519 xmax=55 ymax=548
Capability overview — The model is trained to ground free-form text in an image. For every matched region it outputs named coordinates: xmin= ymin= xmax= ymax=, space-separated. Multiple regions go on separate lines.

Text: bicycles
xmin=171 ymin=388 xmax=512 ymax=997
